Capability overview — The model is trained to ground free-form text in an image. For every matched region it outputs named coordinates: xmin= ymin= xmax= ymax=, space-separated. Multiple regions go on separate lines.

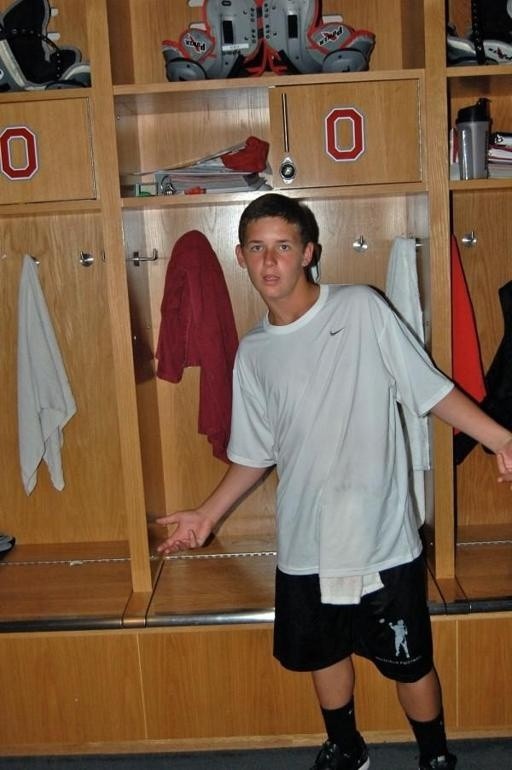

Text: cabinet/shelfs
xmin=0 ymin=0 xmax=512 ymax=758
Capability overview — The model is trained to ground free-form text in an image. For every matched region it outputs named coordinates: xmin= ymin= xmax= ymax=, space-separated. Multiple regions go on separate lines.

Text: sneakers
xmin=306 ymin=731 xmax=370 ymax=770
xmin=418 ymin=752 xmax=458 ymax=770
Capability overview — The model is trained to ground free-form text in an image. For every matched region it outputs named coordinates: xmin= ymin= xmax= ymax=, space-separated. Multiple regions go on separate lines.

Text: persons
xmin=155 ymin=192 xmax=511 ymax=770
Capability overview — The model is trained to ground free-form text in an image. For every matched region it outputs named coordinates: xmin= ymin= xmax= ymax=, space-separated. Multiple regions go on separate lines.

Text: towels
xmin=450 ymin=231 xmax=489 ymax=437
xmin=150 ymin=230 xmax=244 ymax=465
xmin=380 ymin=235 xmax=429 ymax=538
xmin=14 ymin=255 xmax=82 ymax=495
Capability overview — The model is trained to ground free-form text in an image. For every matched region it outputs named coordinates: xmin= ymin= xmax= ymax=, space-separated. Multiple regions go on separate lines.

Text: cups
xmin=455 ymin=97 xmax=491 ymax=181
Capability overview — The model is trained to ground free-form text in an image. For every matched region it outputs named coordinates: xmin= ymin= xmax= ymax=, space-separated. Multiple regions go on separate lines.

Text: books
xmin=485 ymin=131 xmax=512 ymax=179
